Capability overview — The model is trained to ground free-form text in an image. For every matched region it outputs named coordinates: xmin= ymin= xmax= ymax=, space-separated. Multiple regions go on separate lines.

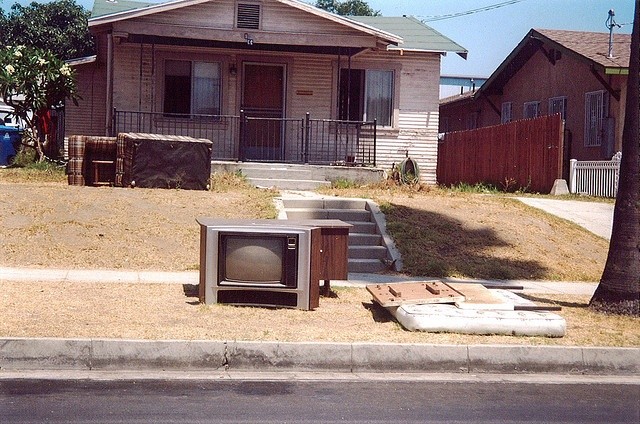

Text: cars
xmin=0 ymin=127 xmax=27 ymax=167
xmin=0 ymin=104 xmax=26 ymax=129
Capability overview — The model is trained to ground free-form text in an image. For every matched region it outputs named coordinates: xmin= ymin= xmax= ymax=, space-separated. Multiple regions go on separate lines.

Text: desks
xmin=193 ymin=215 xmax=354 ymax=311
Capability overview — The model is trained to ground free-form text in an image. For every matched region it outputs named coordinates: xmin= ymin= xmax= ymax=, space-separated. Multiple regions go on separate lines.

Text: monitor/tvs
xmin=202 ymin=227 xmax=311 ymax=310
